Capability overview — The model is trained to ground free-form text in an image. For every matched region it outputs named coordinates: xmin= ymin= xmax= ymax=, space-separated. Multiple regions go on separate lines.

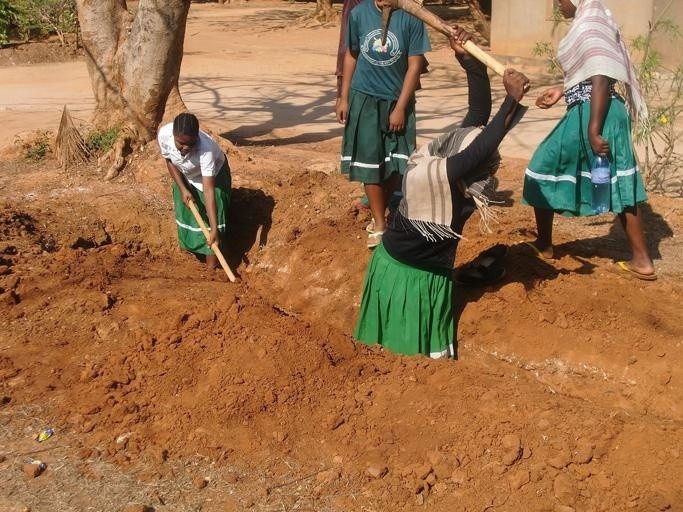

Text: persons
xmin=157 ymin=111 xmax=233 ymax=269
xmin=335 ymin=0 xmax=432 ymax=249
xmin=354 ymin=23 xmax=529 ymax=363
xmin=515 ymin=0 xmax=659 ymax=281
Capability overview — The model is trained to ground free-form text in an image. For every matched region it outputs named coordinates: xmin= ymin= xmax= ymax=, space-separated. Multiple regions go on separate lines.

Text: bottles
xmin=590 ymin=153 xmax=612 ymax=215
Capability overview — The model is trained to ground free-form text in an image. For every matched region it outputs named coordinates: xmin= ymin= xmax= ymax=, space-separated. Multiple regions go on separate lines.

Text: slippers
xmin=511 ymin=242 xmax=556 ymax=264
xmin=366 ymin=217 xmax=387 ymax=249
xmin=607 ymin=261 xmax=658 ymax=280
